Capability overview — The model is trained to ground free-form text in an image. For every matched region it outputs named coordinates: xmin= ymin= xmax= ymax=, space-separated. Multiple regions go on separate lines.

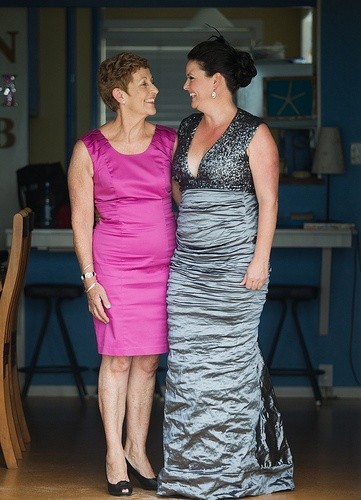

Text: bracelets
xmin=81 ymin=264 xmax=97 ymax=292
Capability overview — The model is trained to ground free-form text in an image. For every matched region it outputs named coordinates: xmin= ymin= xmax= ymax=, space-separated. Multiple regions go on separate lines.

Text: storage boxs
xmin=251 ymin=44 xmax=287 ymax=64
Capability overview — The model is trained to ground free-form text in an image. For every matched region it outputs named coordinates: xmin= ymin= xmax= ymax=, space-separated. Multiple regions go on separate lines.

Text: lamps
xmin=301 ymin=124 xmax=357 ymax=230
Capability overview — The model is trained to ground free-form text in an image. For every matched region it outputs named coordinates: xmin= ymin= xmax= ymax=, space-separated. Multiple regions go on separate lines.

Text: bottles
xmin=37 ymin=181 xmax=55 ymax=227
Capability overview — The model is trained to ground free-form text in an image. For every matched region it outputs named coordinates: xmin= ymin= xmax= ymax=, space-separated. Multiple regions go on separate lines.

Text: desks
xmin=4 ymin=228 xmax=352 ymax=404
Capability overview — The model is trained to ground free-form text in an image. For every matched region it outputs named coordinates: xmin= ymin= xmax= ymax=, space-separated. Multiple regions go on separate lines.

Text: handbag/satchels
xmin=16 ymin=163 xmax=70 ymax=229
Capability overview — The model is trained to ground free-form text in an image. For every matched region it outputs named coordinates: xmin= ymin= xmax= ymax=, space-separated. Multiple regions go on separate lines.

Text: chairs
xmin=0 ymin=206 xmax=34 ymax=471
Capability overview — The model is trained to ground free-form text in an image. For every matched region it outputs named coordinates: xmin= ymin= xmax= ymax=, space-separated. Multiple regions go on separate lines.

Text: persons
xmin=166 ymin=39 xmax=294 ymax=499
xmin=67 ymin=52 xmax=180 ymax=497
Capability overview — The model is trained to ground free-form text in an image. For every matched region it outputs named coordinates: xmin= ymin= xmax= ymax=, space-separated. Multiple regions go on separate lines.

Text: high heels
xmin=105 ymin=453 xmax=133 ymax=496
xmin=125 ymin=457 xmax=157 ymax=491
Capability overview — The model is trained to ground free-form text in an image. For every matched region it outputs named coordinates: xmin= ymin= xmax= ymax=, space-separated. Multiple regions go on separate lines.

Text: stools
xmin=16 ymin=284 xmax=90 ymax=405
xmin=267 ymin=282 xmax=328 ymax=409
